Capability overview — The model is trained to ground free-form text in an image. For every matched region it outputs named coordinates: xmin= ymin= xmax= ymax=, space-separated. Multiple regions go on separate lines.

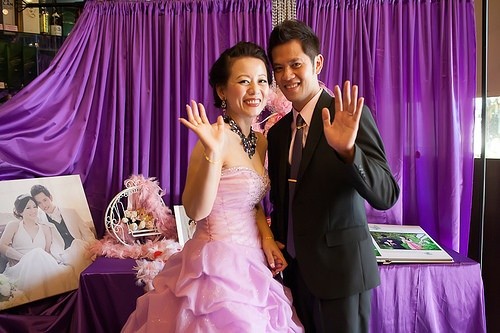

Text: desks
xmin=75 ymin=242 xmax=486 ymax=333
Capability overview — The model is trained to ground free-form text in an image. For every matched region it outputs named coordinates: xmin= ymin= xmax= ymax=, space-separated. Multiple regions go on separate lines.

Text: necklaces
xmin=229 ymin=118 xmax=257 ymax=160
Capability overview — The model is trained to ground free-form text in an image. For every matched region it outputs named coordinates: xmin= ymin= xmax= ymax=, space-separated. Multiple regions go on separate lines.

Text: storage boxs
xmin=18 ymin=0 xmax=40 ymax=34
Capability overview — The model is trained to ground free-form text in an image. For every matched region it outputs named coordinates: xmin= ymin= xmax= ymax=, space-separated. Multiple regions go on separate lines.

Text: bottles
xmin=50 ymin=0 xmax=62 ymax=36
xmin=39 ymin=0 xmax=50 ymax=35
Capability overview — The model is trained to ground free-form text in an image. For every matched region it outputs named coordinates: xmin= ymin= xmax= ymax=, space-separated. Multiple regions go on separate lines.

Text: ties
xmin=287 ymin=114 xmax=304 ymax=259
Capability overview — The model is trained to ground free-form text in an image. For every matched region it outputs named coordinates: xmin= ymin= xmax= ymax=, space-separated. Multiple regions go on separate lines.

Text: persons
xmin=378 ymin=234 xmax=422 ymax=250
xmin=178 ymin=19 xmax=400 ymax=333
xmin=0 ymin=185 xmax=97 ymax=291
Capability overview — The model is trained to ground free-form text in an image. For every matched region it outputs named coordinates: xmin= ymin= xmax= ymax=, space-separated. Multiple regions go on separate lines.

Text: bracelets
xmin=203 ymin=152 xmax=224 ymax=165
xmin=264 ymin=236 xmax=274 ymax=240
